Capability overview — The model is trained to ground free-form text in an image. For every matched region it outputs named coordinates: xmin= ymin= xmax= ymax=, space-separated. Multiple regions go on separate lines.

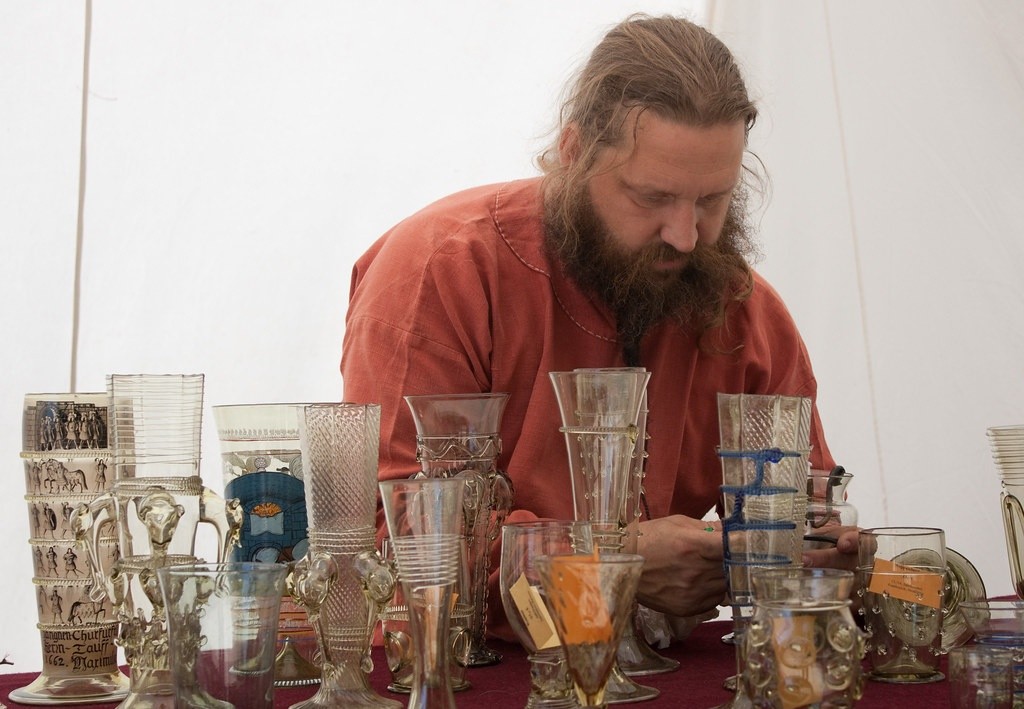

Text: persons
xmin=339 ymin=12 xmax=880 ymax=646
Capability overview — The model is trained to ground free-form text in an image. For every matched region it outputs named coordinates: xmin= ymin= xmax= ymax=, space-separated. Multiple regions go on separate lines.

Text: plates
xmin=880 ymin=547 xmax=987 ymax=654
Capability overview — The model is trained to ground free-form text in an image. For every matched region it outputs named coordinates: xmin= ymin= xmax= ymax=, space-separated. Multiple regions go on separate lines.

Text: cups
xmin=157 ymin=562 xmax=287 ymax=709
xmin=535 ymin=553 xmax=645 ymax=709
xmin=213 ymin=402 xmax=323 ymax=686
xmin=380 ymin=480 xmax=465 ymax=709
xmin=949 ymin=645 xmax=1013 ymax=709
xmin=7 ymin=392 xmax=131 ymax=704
xmin=748 ymin=568 xmax=859 ymax=709
xmin=986 ymin=425 xmax=1024 ymax=601
xmin=383 ymin=539 xmax=474 ymax=693
xmin=856 ymin=566 xmax=941 ymax=678
xmin=959 ymin=601 xmax=1024 ymax=709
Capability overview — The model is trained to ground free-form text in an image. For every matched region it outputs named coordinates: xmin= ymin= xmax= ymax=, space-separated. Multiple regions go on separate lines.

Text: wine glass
xmin=289 ymin=404 xmax=403 ymax=709
xmin=574 ymin=369 xmax=679 ymax=676
xmin=549 ymin=371 xmax=658 ymax=704
xmin=858 ymin=527 xmax=945 ymax=684
xmin=404 ymin=394 xmax=515 ymax=667
xmin=718 ymin=394 xmax=811 ymax=692
xmin=70 ymin=375 xmax=243 ymax=709
xmin=500 ymin=520 xmax=579 ymax=709
